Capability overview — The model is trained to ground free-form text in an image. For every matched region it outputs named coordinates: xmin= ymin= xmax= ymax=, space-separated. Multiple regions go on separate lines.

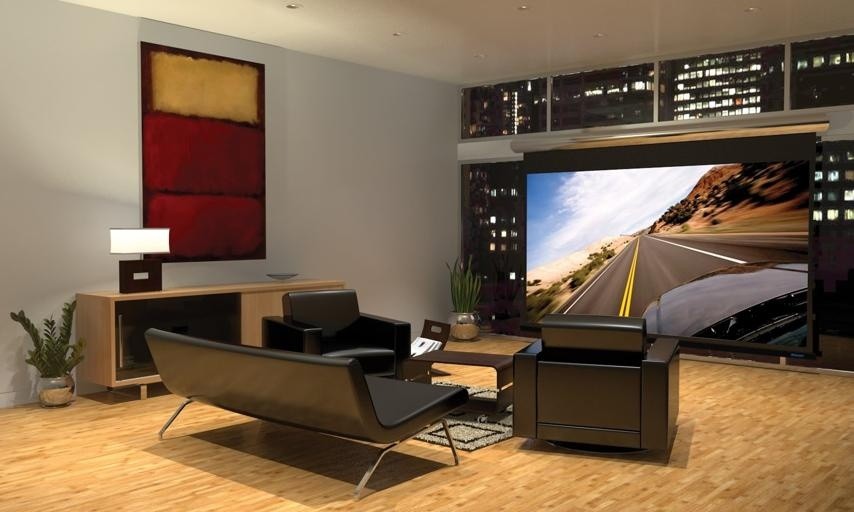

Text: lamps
xmin=507 ymin=108 xmax=831 ymax=157
xmin=105 ymin=225 xmax=174 ymax=297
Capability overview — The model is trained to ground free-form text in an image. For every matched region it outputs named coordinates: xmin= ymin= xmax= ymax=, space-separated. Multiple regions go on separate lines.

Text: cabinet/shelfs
xmin=75 ymin=276 xmax=346 ymax=402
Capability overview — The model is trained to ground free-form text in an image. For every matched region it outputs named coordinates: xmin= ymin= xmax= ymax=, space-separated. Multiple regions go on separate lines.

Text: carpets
xmin=406 ymin=377 xmax=514 ymax=453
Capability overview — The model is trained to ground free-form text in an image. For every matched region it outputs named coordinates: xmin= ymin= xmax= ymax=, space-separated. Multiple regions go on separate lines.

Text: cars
xmin=635 ymin=258 xmax=808 ymax=346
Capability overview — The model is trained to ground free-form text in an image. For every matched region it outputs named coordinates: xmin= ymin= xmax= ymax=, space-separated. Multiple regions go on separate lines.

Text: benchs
xmin=141 ymin=324 xmax=472 ymax=501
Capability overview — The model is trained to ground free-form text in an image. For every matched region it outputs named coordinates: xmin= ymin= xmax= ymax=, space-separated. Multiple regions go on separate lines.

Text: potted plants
xmin=442 ymin=252 xmax=483 ymax=343
xmin=8 ymin=298 xmax=90 ymax=411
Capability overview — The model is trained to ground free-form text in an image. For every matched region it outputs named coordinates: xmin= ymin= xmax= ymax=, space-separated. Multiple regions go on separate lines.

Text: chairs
xmin=508 ymin=307 xmax=685 ymax=461
xmin=257 ymin=287 xmax=415 ymax=381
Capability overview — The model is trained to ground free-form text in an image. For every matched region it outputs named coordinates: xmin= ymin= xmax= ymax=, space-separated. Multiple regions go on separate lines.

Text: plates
xmin=266 ymin=272 xmax=299 ymax=280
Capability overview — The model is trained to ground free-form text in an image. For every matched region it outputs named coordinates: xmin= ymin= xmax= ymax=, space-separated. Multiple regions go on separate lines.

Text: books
xmin=411 ymin=336 xmax=442 ymax=358
